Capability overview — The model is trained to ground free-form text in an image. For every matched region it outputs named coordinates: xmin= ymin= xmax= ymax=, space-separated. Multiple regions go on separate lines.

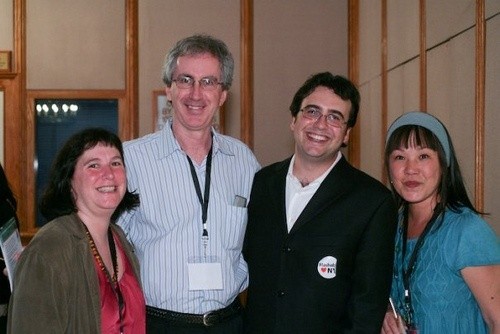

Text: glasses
xmin=171 ymin=75 xmax=224 ymax=89
xmin=299 ymin=108 xmax=349 ymax=128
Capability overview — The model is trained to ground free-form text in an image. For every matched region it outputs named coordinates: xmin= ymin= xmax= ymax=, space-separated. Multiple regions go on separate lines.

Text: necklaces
xmin=82 ymin=221 xmax=127 ymax=333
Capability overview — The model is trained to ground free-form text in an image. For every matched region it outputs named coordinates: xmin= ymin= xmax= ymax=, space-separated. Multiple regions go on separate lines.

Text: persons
xmin=243 ymin=70 xmax=396 ymax=334
xmin=7 ymin=127 xmax=147 ymax=334
xmin=115 ymin=34 xmax=262 ymax=334
xmin=385 ymin=112 xmax=500 ymax=334
